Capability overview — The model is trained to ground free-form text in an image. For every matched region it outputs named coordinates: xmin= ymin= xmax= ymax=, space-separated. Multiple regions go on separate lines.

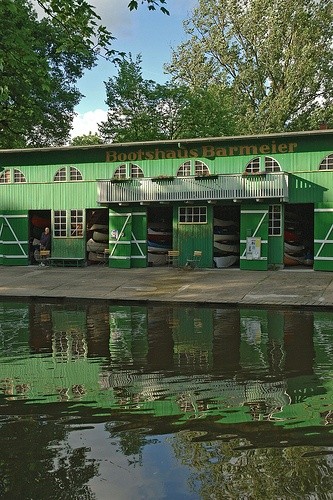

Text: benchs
xmin=46 ymin=257 xmax=84 ymax=268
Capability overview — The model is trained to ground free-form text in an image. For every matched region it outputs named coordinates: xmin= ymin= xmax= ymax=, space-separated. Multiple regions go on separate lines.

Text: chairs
xmin=35 ymin=250 xmax=50 ymax=270
xmin=168 ymin=251 xmax=180 ymax=270
xmin=96 ymin=248 xmax=109 ymax=268
xmin=186 ymin=250 xmax=202 ymax=271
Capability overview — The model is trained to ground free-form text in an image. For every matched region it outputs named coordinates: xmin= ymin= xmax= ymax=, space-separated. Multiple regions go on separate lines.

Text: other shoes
xmin=40 ymin=262 xmax=46 ymax=267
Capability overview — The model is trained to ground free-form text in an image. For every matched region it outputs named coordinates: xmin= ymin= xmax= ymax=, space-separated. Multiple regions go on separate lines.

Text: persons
xmin=39 ymin=228 xmax=51 ymax=267
